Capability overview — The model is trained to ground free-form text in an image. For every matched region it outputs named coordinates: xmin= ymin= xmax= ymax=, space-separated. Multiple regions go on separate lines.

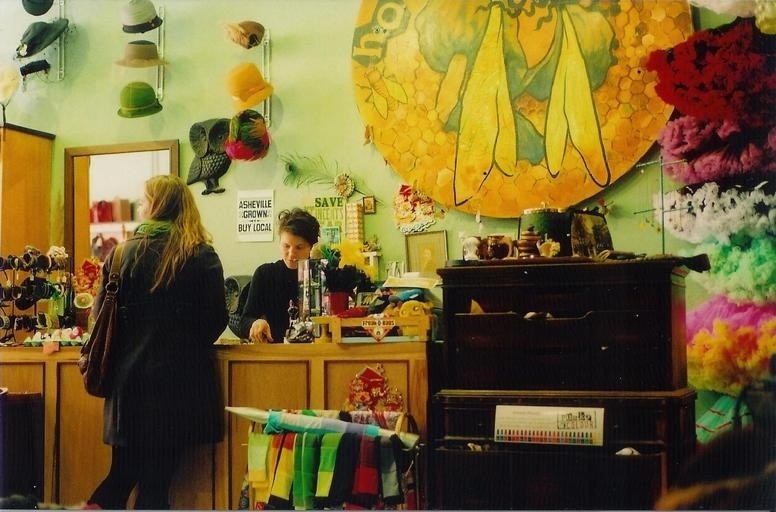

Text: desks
xmin=0 ymin=343 xmax=426 ymax=511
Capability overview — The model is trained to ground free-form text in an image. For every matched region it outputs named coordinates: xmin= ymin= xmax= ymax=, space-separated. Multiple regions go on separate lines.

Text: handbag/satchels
xmin=82 ymin=240 xmax=124 ymax=397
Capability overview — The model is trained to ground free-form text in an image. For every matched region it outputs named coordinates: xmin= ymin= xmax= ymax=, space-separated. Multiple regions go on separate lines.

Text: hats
xmin=22 ymin=0 xmax=54 ymax=16
xmin=16 ymin=18 xmax=68 ymax=56
xmin=117 ymin=83 xmax=163 ymax=118
xmin=226 ymin=63 xmax=273 ymax=110
xmin=115 ymin=40 xmax=170 ymax=68
xmin=120 ymin=1 xmax=163 ymax=34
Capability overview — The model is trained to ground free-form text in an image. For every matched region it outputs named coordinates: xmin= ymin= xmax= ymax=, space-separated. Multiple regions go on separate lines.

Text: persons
xmin=81 ymin=174 xmax=229 ymax=511
xmin=228 ymin=208 xmax=321 ymax=341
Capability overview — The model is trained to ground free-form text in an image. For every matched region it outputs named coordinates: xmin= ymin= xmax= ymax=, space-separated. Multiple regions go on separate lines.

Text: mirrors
xmin=64 ymin=139 xmax=179 ymax=286
